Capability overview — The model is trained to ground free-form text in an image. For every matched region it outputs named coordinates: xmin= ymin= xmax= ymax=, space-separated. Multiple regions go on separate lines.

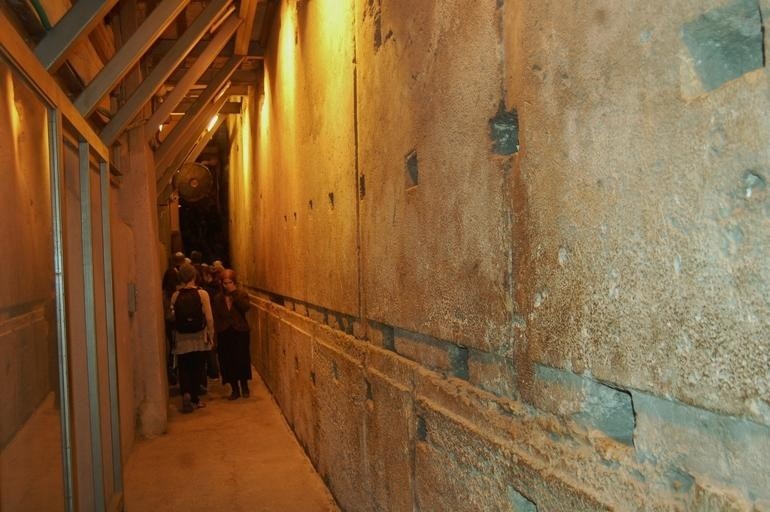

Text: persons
xmin=162 ymin=251 xmax=252 ymax=411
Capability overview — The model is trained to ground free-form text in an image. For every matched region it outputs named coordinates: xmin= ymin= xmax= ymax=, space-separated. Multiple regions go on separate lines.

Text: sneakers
xmin=178 ymin=404 xmax=194 ymax=413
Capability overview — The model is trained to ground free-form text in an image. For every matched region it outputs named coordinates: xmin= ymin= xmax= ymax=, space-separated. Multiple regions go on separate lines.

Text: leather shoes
xmin=228 ymin=389 xmax=240 ymax=400
xmin=241 ymin=388 xmax=250 ymax=398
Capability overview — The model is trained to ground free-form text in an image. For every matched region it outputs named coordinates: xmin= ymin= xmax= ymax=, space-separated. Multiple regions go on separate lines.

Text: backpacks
xmin=172 ymin=285 xmax=207 ymax=334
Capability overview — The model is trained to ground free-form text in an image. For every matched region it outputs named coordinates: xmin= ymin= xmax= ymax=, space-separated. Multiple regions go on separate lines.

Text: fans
xmin=174 ymin=157 xmax=217 ymax=204
